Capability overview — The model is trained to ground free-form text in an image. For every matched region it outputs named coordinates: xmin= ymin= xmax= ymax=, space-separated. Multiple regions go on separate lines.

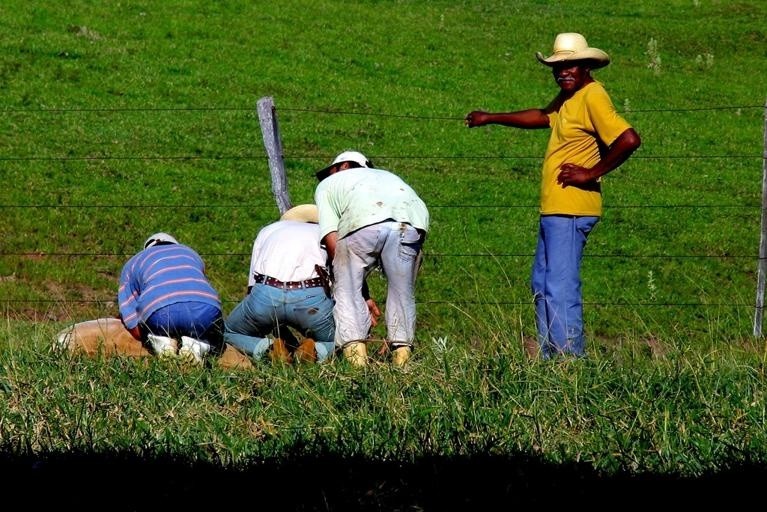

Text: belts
xmin=255 ymin=272 xmax=324 ymax=291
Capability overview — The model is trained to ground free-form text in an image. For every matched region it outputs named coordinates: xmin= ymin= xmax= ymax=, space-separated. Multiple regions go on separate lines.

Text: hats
xmin=535 ymin=32 xmax=610 ymax=69
xmin=143 ymin=232 xmax=179 ymax=251
xmin=280 ymin=203 xmax=320 ymax=224
xmin=316 ymin=150 xmax=373 ymax=182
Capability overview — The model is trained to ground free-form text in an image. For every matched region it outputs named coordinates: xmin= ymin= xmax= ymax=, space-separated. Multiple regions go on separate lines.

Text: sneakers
xmin=148 ymin=334 xmax=211 ymax=363
xmin=343 ymin=342 xmax=368 ymax=365
xmin=272 ymin=338 xmax=315 ymax=366
xmin=390 ymin=347 xmax=411 ymax=368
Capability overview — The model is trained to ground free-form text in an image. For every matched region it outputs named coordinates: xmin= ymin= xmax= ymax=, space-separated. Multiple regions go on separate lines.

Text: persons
xmin=465 ymin=33 xmax=641 ymax=361
